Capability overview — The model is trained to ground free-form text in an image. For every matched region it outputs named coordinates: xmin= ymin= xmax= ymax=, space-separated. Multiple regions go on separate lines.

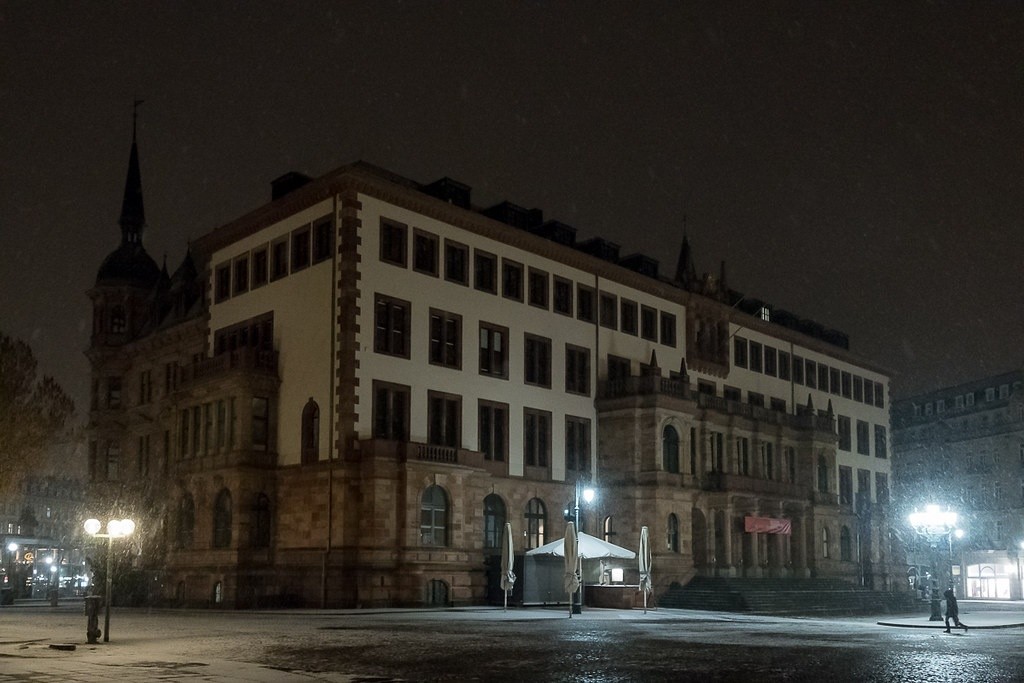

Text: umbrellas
xmin=524 ymin=531 xmax=636 ymax=605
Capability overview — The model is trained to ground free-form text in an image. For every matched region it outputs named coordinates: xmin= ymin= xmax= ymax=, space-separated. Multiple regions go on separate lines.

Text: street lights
xmin=909 ymin=503 xmax=956 ymax=621
xmin=84 ymin=519 xmax=136 ymax=642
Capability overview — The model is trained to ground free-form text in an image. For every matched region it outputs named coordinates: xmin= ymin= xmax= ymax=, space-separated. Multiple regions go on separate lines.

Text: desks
xmin=585 ymin=584 xmax=654 ymax=610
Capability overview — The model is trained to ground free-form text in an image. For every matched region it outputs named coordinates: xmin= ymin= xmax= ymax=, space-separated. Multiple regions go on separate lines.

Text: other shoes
xmin=965 ymin=626 xmax=968 ymax=631
xmin=944 ymin=629 xmax=950 ymax=632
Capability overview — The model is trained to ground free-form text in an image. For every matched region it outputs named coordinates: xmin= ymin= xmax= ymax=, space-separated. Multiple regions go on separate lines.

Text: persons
xmin=943 ymin=590 xmax=968 ymax=633
